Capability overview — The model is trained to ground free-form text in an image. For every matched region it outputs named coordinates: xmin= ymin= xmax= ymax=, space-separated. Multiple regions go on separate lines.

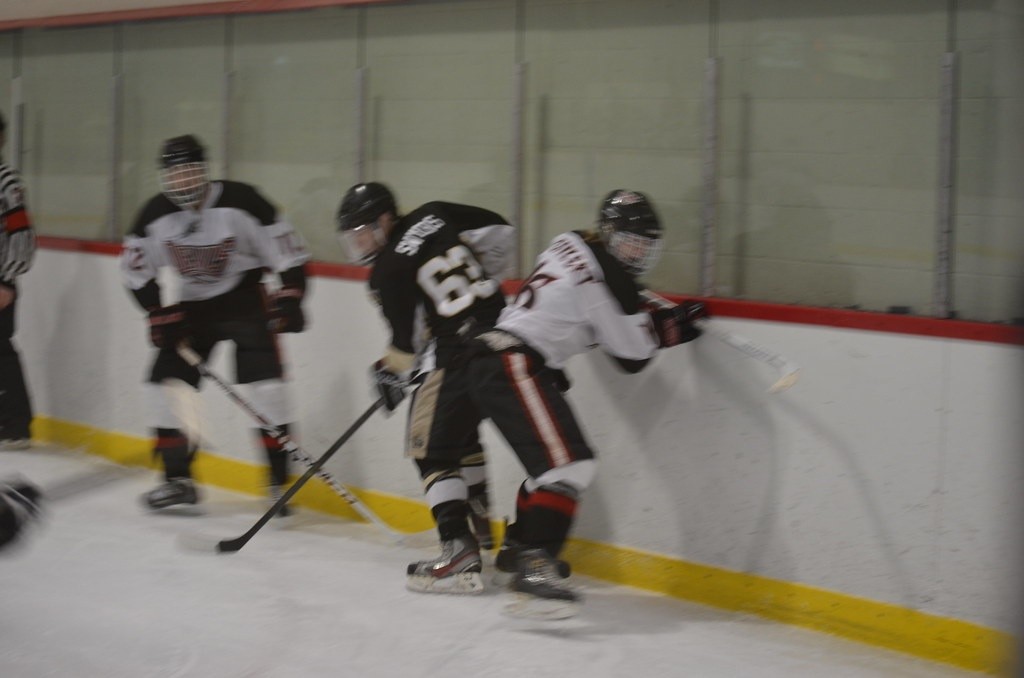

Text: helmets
xmin=597 ymin=188 xmax=665 ymax=280
xmin=336 ymin=182 xmax=397 ymax=271
xmin=156 ymin=136 xmax=210 ymax=210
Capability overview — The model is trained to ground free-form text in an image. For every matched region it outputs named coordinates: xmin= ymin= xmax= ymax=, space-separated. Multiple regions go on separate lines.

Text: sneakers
xmin=145 ymin=446 xmax=201 ymax=516
xmin=472 ymin=515 xmax=494 ymax=548
xmin=494 ymin=562 xmax=581 ymax=619
xmin=268 ymin=449 xmax=298 ymax=527
xmin=0 ymin=416 xmax=34 ymax=450
xmin=492 ymin=516 xmax=572 ymax=588
xmin=405 ymin=534 xmax=485 ymax=596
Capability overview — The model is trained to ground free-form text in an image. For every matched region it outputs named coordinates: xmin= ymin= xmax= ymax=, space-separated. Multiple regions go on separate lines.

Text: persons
xmin=119 ymin=135 xmax=312 ymax=520
xmin=472 ymin=188 xmax=712 ymax=604
xmin=0 ymin=111 xmax=37 ymax=451
xmin=336 ymin=182 xmax=516 ymax=596
xmin=0 ymin=483 xmax=44 ymax=549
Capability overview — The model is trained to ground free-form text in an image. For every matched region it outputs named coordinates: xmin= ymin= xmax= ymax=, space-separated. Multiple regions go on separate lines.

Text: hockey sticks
xmin=636 ymin=289 xmax=799 ymax=404
xmin=173 ymin=334 xmax=458 ymax=554
xmin=0 ymin=433 xmax=184 ymax=524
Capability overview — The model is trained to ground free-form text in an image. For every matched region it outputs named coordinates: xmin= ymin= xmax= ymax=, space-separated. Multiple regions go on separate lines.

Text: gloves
xmin=370 ymin=359 xmax=405 ymax=410
xmin=644 ymin=300 xmax=708 ymax=348
xmin=148 ymin=302 xmax=191 ymax=351
xmin=266 ymin=288 xmax=305 ymax=332
